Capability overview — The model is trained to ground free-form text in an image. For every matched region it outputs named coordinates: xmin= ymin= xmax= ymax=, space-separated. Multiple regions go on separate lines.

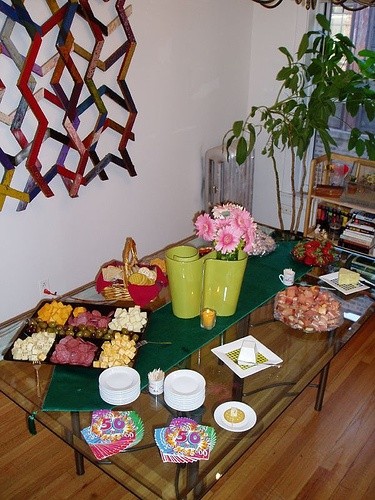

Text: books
xmin=338 ymin=212 xmax=375 ymax=254
xmin=316 ymin=201 xmax=352 ymax=243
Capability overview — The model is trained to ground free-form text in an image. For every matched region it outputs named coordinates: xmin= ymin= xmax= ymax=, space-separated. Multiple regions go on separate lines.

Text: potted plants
xmin=221 ymin=12 xmax=375 ymax=244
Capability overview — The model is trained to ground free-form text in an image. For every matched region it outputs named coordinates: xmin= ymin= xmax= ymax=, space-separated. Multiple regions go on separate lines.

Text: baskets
xmin=96 ymin=237 xmax=169 ymax=304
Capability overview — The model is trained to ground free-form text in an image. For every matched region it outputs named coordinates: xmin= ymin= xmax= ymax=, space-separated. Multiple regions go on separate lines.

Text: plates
xmin=99 ymin=366 xmax=142 ymax=406
xmin=274 ymin=286 xmax=345 ymax=335
xmin=163 ymin=369 xmax=207 ymax=412
xmin=214 ymin=400 xmax=257 ymax=433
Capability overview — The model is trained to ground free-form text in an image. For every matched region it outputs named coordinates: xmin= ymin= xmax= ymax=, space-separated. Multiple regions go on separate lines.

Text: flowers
xmin=194 ymin=200 xmax=277 ymax=262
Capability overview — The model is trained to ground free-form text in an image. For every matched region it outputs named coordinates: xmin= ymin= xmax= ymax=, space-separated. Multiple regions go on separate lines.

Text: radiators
xmin=201 ymin=142 xmax=256 ymax=221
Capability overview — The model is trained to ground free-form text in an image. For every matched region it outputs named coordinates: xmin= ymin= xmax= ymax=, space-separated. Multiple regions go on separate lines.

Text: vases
xmin=164 ymin=244 xmax=249 ymax=321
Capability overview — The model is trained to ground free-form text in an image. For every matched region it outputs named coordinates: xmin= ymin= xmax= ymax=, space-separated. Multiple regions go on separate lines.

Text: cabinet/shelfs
xmin=303 ymin=152 xmax=374 ymax=295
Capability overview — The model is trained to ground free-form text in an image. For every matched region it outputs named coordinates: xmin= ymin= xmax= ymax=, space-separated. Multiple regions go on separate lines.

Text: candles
xmin=200 ymin=307 xmax=217 ymax=330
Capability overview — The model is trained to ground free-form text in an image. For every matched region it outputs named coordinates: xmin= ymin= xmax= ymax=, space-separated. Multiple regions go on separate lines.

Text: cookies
xmin=129 ymin=267 xmax=155 ymax=286
xmin=224 ymin=408 xmax=244 ymax=423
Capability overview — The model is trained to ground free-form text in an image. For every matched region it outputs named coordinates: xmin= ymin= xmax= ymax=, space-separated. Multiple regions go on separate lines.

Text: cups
xmin=147 ymin=373 xmax=164 ymax=396
xmin=279 ymin=269 xmax=296 ymax=286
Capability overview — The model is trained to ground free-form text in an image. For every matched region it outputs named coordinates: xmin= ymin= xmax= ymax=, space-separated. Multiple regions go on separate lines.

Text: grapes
xmin=25 ymin=319 xmax=139 ymax=341
